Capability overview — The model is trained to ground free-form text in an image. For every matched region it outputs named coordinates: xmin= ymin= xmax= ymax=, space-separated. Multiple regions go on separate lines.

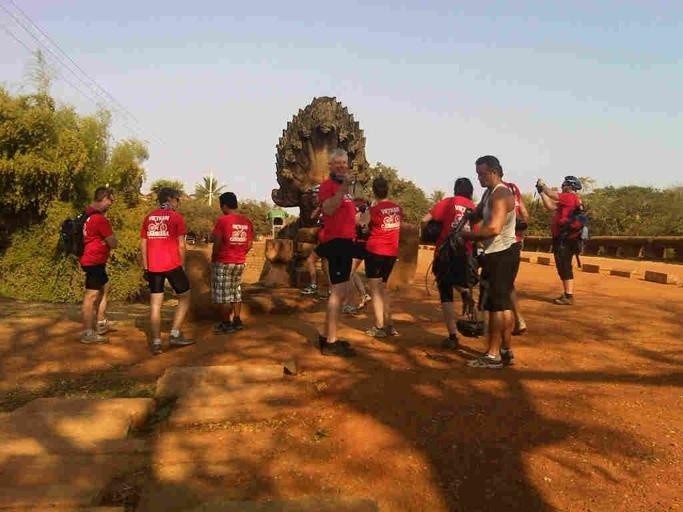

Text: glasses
xmin=170 ymin=196 xmax=180 ymax=201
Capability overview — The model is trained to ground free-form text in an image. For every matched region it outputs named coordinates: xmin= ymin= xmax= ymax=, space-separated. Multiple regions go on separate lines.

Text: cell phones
xmin=352 ymin=162 xmax=358 ymax=171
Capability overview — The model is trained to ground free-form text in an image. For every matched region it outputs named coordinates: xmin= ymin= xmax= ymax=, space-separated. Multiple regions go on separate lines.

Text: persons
xmin=420 ymin=155 xmax=527 ymax=370
xmin=536 ymin=176 xmax=582 ymax=304
xmin=78 ymin=186 xmax=119 ymax=344
xmin=300 ymin=147 xmax=403 ymax=357
xmin=139 ymin=188 xmax=196 ymax=355
xmin=209 ymin=191 xmax=254 ymax=335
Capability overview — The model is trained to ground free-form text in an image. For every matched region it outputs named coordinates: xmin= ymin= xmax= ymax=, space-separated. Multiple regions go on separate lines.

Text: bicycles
xmin=424 ymin=259 xmax=440 ymax=300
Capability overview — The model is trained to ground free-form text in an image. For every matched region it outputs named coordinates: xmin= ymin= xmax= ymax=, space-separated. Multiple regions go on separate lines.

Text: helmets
xmin=565 ymin=175 xmax=583 ymax=189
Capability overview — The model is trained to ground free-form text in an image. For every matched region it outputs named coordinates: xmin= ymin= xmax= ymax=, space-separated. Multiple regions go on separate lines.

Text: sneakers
xmin=318 ymin=333 xmax=357 ymax=358
xmin=364 ymin=324 xmax=401 ymax=338
xmin=551 ymin=291 xmax=578 ymax=306
xmin=510 ymin=318 xmax=529 ymax=337
xmin=168 ymin=331 xmax=195 ymax=346
xmin=78 ymin=330 xmax=112 ymax=346
xmin=464 ymin=346 xmax=517 ymax=369
xmin=342 ymin=293 xmax=373 ymax=314
xmin=300 ymin=281 xmax=333 ymax=299
xmin=440 ymin=334 xmax=459 ymax=350
xmin=150 ymin=339 xmax=163 ymax=354
xmin=95 ymin=319 xmax=121 ymax=334
xmin=210 ymin=315 xmax=246 ymax=335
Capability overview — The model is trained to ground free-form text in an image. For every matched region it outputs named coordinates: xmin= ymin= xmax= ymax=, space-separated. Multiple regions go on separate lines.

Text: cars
xmin=186 ymin=235 xmax=214 ymax=245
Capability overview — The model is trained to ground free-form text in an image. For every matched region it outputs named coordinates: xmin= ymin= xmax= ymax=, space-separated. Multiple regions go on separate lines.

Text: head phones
xmin=329 ymin=172 xmax=344 ymax=184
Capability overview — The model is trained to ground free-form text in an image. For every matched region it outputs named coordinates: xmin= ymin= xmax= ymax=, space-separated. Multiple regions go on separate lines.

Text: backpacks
xmin=551 ymin=190 xmax=590 ymax=256
xmin=431 ymin=208 xmax=481 ymax=289
